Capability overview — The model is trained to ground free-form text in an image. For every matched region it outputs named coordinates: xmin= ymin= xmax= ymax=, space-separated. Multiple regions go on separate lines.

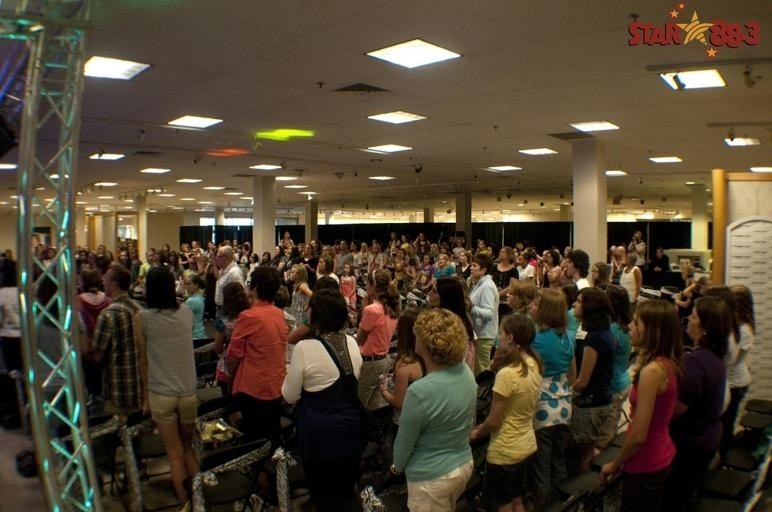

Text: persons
xmin=2 ymin=231 xmax=755 ymax=512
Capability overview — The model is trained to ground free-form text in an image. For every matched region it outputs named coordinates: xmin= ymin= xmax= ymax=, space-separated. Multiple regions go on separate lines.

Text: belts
xmin=361 ymin=353 xmax=388 ymax=362
